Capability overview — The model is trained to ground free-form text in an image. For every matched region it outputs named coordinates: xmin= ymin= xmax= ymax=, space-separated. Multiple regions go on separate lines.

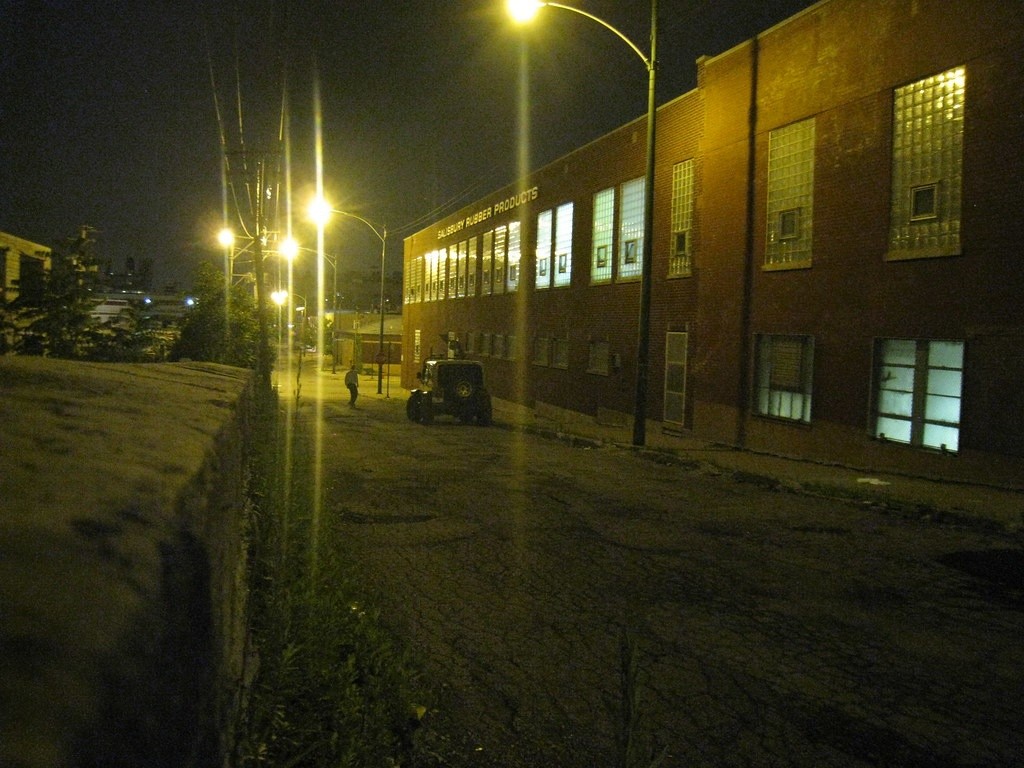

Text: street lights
xmin=280 ymin=237 xmax=338 ymax=374
xmin=271 ymin=290 xmax=307 ymax=358
xmin=506 ymin=0 xmax=658 ymax=447
xmin=308 ymin=198 xmax=387 ymax=394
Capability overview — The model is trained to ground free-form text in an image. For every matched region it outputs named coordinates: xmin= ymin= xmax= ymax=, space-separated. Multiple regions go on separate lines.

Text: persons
xmin=345 ymin=365 xmax=358 ymax=408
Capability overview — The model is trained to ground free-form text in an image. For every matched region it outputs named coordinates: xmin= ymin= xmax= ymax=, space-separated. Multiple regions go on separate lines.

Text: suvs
xmin=406 ymin=360 xmax=493 ymax=427
